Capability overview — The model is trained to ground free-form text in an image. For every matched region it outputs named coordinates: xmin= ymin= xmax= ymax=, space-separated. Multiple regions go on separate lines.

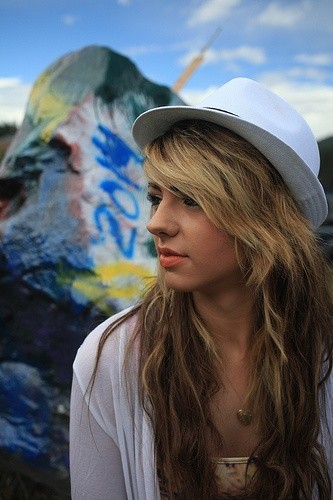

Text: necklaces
xmin=209 ymin=350 xmax=261 ymax=426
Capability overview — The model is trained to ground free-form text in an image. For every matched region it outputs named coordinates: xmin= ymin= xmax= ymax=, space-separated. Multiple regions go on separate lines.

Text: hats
xmin=130 ymin=74 xmax=327 ymax=228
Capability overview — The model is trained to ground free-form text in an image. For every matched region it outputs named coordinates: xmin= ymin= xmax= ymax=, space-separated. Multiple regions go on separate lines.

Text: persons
xmin=65 ymin=77 xmax=333 ymax=500
